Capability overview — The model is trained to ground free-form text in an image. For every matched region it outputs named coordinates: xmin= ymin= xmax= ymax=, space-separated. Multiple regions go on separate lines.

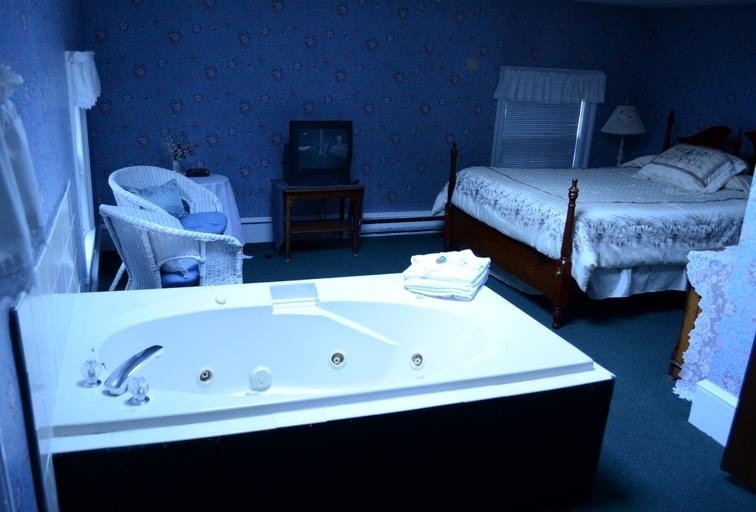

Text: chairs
xmin=98 ymin=165 xmax=244 ymax=290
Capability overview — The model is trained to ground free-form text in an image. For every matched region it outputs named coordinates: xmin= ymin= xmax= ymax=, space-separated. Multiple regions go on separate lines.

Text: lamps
xmin=601 ymin=97 xmax=648 ymax=166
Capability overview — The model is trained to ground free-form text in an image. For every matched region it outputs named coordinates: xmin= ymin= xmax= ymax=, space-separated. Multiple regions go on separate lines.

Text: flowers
xmin=158 ymin=132 xmax=195 ymax=160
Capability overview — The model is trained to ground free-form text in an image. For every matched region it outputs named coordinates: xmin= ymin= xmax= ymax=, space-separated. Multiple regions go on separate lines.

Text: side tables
xmin=179 ymin=172 xmax=245 ymax=248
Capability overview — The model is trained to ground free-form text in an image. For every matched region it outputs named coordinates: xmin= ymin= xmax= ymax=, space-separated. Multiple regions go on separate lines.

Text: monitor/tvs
xmin=282 ymin=119 xmax=354 ymax=186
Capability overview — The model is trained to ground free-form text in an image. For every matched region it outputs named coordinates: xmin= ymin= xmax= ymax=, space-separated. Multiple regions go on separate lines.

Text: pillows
xmin=119 ymin=178 xmax=194 ymax=220
xmin=626 ymin=143 xmax=749 ymax=194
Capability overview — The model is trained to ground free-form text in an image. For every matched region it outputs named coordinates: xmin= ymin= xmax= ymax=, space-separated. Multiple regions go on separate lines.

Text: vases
xmin=172 ymin=159 xmax=180 ymax=173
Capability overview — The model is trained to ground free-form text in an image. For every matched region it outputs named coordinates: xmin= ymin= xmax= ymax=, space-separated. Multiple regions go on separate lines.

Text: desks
xmin=270 ymin=175 xmax=365 ymax=263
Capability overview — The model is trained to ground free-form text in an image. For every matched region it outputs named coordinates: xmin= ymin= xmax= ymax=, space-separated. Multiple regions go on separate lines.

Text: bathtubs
xmin=52 ymin=273 xmax=593 ymax=435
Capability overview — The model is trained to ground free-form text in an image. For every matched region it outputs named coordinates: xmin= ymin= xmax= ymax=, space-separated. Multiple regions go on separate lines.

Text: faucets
xmin=104 ymin=346 xmax=164 ymax=396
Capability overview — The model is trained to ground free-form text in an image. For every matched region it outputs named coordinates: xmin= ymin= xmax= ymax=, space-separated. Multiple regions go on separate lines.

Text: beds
xmin=444 ymin=107 xmax=756 ymax=329
